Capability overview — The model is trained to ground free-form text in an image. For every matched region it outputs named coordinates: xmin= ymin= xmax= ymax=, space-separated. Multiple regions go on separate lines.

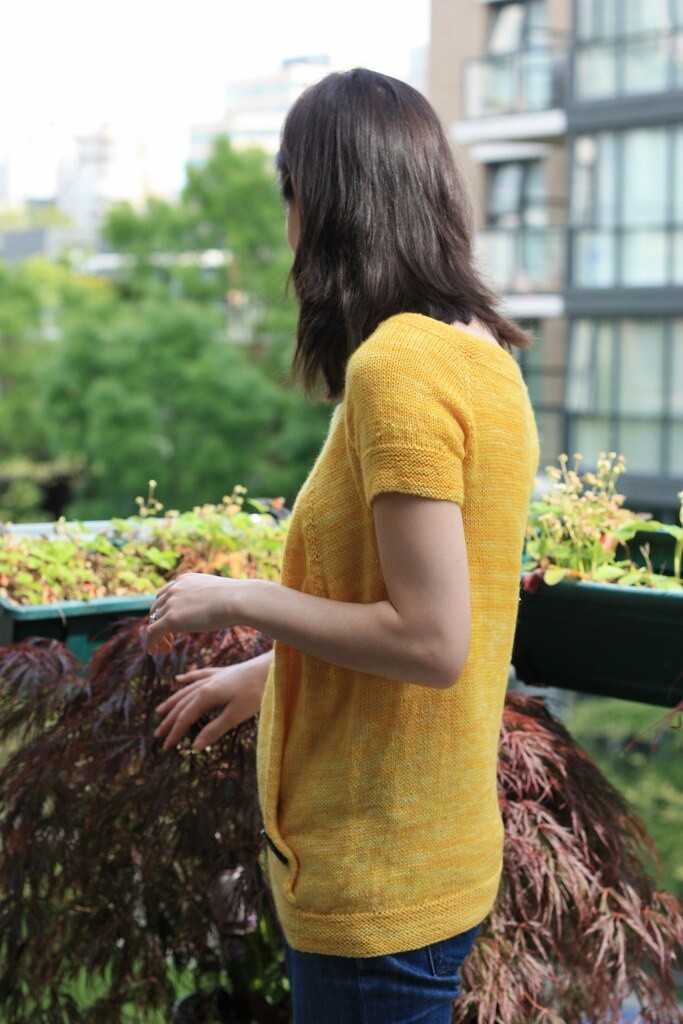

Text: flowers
xmin=0 ymin=480 xmax=291 ymax=626
xmin=522 ymin=452 xmax=682 ymax=594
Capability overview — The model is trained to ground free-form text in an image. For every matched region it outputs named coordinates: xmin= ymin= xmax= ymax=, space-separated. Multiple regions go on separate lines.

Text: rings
xmin=149 ymin=613 xmax=158 ymax=622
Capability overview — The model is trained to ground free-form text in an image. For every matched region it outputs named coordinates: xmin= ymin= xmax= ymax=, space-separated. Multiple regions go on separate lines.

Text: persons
xmin=144 ymin=69 xmax=542 ymax=1023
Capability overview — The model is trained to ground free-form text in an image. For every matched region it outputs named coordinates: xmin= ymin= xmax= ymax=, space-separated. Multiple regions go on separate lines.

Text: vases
xmin=0 ymin=516 xmax=277 ymax=677
xmin=511 ymin=531 xmax=683 ymax=709
